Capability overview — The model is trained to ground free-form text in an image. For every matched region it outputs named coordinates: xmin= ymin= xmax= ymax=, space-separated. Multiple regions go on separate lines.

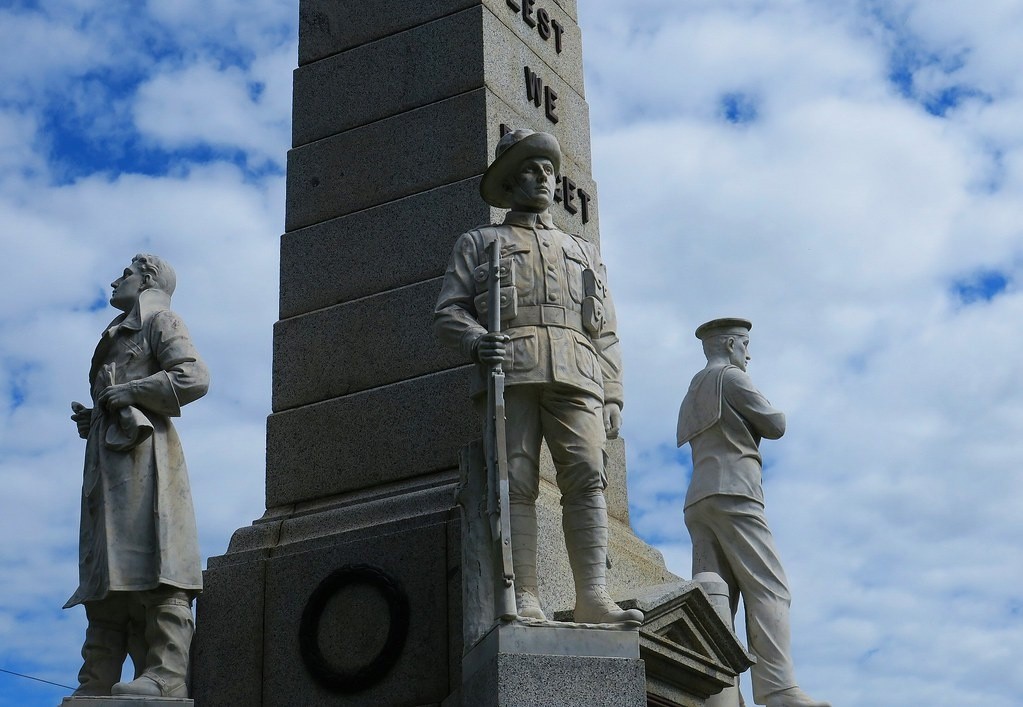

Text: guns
xmin=482 ymin=237 xmax=521 ymax=621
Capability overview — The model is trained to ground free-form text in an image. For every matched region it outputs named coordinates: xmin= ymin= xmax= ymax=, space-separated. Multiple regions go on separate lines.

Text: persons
xmin=677 ymin=317 xmax=833 ymax=707
xmin=433 ymin=130 xmax=650 ymax=627
xmin=62 ymin=253 xmax=209 ymax=700
